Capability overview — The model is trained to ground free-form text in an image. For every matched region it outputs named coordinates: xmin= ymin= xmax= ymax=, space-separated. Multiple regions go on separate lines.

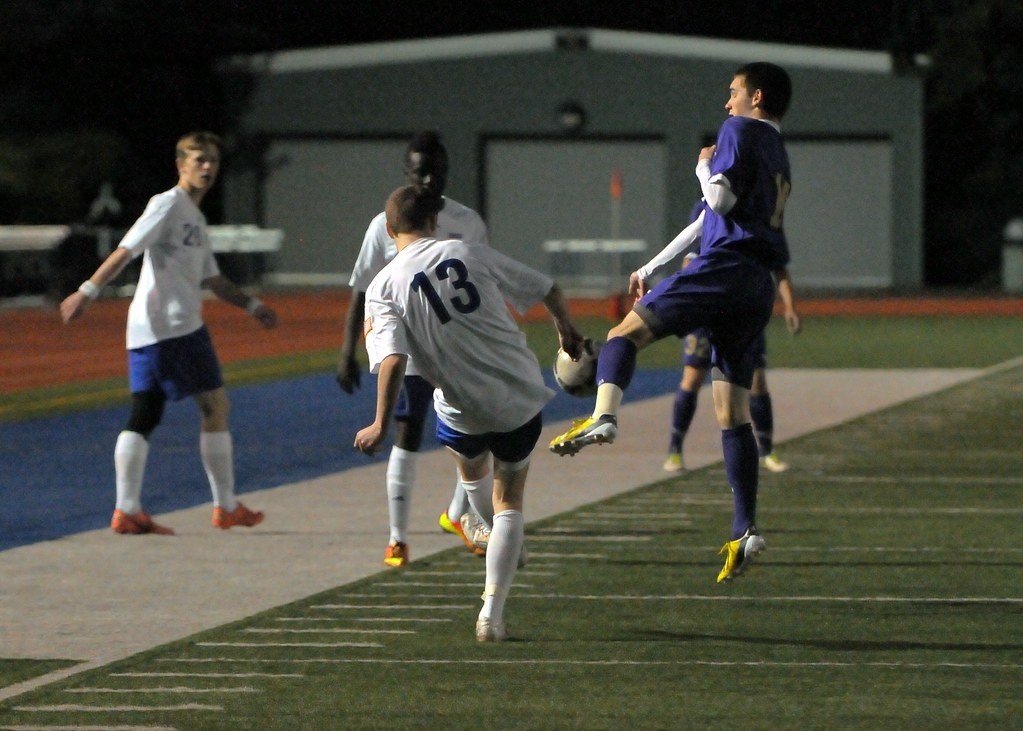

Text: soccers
xmin=552 ymin=335 xmax=603 ymax=399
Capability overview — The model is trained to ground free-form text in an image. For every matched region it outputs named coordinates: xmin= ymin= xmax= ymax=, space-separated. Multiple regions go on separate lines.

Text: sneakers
xmin=549 ymin=414 xmax=617 ymax=457
xmin=439 ymin=509 xmax=486 ymax=556
xmin=717 ymin=526 xmax=766 ymax=584
xmin=663 ymin=453 xmax=686 ymax=472
xmin=384 ymin=542 xmax=409 ymax=567
xmin=459 ymin=512 xmax=528 ymax=568
xmin=112 ymin=509 xmax=175 ymax=537
xmin=212 ymin=502 xmax=264 ymax=529
xmin=475 ymin=617 xmax=509 ymax=642
xmin=759 ymin=452 xmax=792 ymax=472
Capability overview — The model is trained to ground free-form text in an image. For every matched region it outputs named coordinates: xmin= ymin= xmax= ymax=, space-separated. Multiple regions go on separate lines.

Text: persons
xmin=352 ymin=182 xmax=585 ymax=644
xmin=59 ymin=134 xmax=276 ymax=535
xmin=549 ymin=64 xmax=792 ymax=584
xmin=666 ymin=200 xmax=802 ymax=473
xmin=337 ymin=130 xmax=489 ymax=566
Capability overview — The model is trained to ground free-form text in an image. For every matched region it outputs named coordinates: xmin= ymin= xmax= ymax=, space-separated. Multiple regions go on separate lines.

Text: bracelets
xmin=247 ymin=297 xmax=261 ymax=314
xmin=79 ymin=280 xmax=100 ymax=300
